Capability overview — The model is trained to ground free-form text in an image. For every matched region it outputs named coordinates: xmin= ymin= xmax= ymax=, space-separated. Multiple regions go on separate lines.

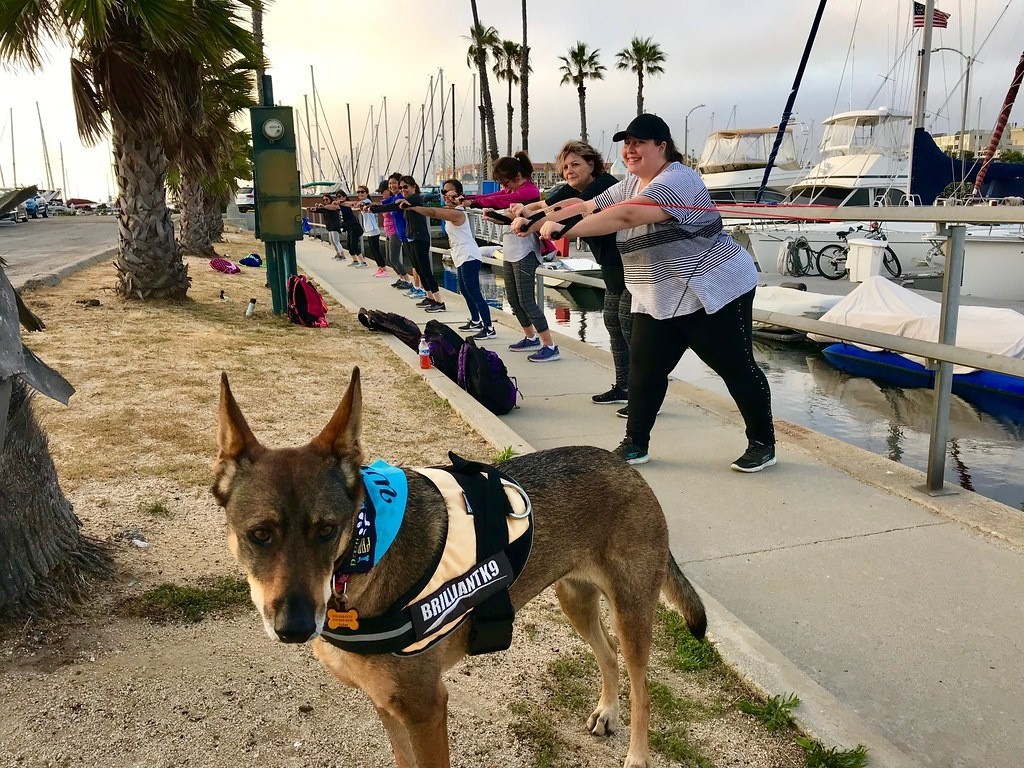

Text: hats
xmin=612 ymin=114 xmax=671 ymax=143
xmin=375 ymin=180 xmax=388 ymax=192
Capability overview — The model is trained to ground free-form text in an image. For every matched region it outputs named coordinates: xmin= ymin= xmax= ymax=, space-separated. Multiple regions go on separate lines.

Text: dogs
xmin=211 ymin=366 xmax=709 ymax=768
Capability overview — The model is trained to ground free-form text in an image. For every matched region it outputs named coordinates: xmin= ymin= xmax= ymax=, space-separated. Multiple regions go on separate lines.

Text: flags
xmin=914 ymin=1 xmax=950 ymax=29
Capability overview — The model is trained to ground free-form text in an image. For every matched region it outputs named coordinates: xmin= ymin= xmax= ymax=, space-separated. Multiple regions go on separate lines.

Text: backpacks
xmin=358 ymin=307 xmax=423 ymax=352
xmin=424 ymin=319 xmax=467 ymax=381
xmin=457 ymin=336 xmax=523 ymax=415
xmin=285 ymin=274 xmax=328 ymax=329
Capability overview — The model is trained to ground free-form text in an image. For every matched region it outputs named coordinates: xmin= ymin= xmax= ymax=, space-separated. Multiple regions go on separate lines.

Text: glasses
xmin=336 ymin=195 xmax=341 ymax=198
xmin=503 ymin=180 xmax=510 ymax=188
xmin=440 ymin=189 xmax=447 ymax=195
xmin=356 ymin=190 xmax=366 ymax=193
xmin=322 ymin=199 xmax=328 ymax=201
xmin=397 ymin=185 xmax=411 ymax=190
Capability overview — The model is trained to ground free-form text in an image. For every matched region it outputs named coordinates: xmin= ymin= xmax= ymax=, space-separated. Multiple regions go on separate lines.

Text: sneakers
xmin=473 ymin=325 xmax=496 ymax=339
xmin=424 ymin=302 xmax=446 ymax=312
xmin=527 ymin=344 xmax=561 ymax=362
xmin=508 ymin=337 xmax=541 ymax=352
xmin=616 ymin=405 xmax=660 ymax=418
xmin=611 ymin=436 xmax=650 ymax=464
xmin=731 ymin=440 xmax=776 ymax=473
xmin=458 ymin=321 xmax=483 ymax=332
xmin=416 ymin=297 xmax=435 ymax=308
xmin=402 ymin=287 xmax=427 ymax=298
xmin=591 ymin=384 xmax=629 ymax=405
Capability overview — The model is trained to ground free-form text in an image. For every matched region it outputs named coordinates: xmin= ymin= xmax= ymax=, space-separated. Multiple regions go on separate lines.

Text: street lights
xmin=684 ymin=104 xmax=706 ymax=166
xmin=931 ymin=45 xmax=971 ymax=159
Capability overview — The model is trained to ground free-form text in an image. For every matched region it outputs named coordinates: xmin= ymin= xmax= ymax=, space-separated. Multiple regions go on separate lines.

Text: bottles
xmin=246 ymin=299 xmax=256 ymax=316
xmin=419 ymin=338 xmax=431 ymax=369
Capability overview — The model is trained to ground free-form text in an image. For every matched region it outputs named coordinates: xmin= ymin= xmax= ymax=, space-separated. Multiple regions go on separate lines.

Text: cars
xmin=0 ymin=187 xmax=49 ymax=222
xmin=234 ymin=187 xmax=256 ymax=212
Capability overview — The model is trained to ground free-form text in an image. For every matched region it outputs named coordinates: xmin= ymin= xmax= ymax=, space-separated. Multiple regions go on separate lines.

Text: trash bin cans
xmin=554 ymin=236 xmax=570 ymax=257
xmin=848 ymin=238 xmax=888 ymax=283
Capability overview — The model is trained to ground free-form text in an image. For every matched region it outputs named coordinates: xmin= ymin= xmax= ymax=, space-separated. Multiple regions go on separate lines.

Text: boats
xmin=694 ymin=126 xmax=810 ymax=206
xmin=776 ymin=107 xmax=913 ymax=206
xmin=493 ymin=248 xmax=603 ymax=287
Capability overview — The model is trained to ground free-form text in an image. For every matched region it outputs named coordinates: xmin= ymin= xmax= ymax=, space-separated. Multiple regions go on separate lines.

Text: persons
xmin=305 ymin=172 xmax=446 ymax=313
xmin=446 ymin=150 xmax=561 ymax=363
xmin=511 ymin=114 xmax=777 ymax=473
xmin=395 ymin=179 xmax=496 ymax=340
xmin=482 ymin=142 xmax=661 ymax=419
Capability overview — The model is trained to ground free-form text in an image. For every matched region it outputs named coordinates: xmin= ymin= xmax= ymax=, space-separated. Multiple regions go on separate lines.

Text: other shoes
xmin=372 ymin=267 xmax=390 ymax=278
xmin=391 ymin=279 xmax=413 ymax=289
xmin=333 ymin=254 xmax=346 ymax=261
xmin=347 ymin=261 xmax=369 ymax=268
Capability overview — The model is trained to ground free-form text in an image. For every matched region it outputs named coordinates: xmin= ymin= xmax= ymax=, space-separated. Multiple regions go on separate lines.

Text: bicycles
xmin=816 ymin=220 xmax=903 ymax=281
